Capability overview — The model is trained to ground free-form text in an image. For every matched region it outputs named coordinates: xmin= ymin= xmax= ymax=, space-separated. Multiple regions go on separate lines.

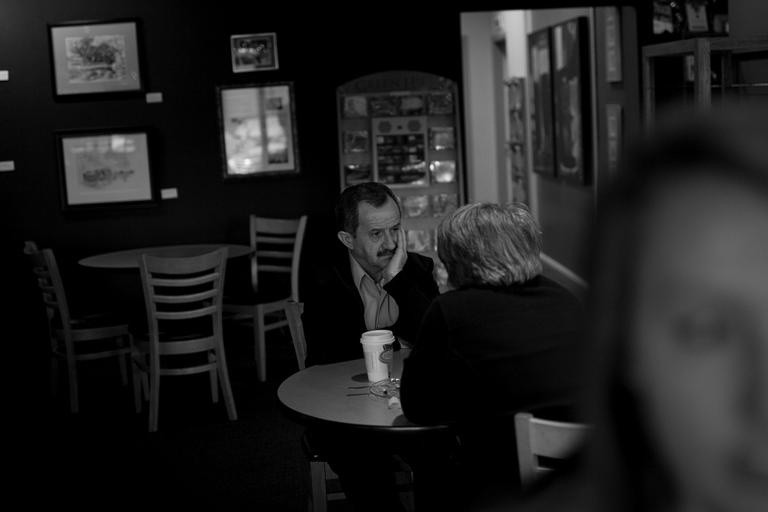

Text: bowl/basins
xmin=367 ymin=379 xmax=400 ymax=415
xmin=378 ymin=346 xmax=412 ymax=378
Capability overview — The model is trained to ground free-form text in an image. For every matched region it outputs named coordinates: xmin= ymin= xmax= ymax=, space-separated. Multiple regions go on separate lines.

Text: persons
xmin=399 ymin=200 xmax=606 ymax=512
xmin=299 ymin=183 xmax=441 ymax=512
xmin=576 ymin=125 xmax=768 ymax=511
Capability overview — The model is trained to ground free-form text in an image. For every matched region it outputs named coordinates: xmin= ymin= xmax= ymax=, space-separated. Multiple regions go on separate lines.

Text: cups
xmin=358 ymin=329 xmax=395 ymax=383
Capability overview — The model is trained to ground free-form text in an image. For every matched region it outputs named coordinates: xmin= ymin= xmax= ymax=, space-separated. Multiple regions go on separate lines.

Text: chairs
xmin=220 ymin=212 xmax=314 ymax=387
xmin=22 ymin=237 xmax=139 ymax=417
xmin=130 ymin=242 xmax=241 ymax=436
xmin=513 ymin=412 xmax=603 ymax=484
xmin=283 ymin=296 xmax=353 ymax=512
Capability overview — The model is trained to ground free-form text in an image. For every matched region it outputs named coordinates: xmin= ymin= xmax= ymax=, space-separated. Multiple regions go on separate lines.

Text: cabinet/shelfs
xmin=640 ymin=38 xmax=767 ymax=140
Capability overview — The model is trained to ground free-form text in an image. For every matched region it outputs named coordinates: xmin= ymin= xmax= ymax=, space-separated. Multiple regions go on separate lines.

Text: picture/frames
xmin=525 ymin=12 xmax=591 ymax=187
xmin=215 ymin=78 xmax=303 ymax=181
xmin=43 ymin=15 xmax=151 ymax=102
xmin=50 ymin=125 xmax=162 ymax=214
xmin=229 ymin=33 xmax=282 ymax=74
